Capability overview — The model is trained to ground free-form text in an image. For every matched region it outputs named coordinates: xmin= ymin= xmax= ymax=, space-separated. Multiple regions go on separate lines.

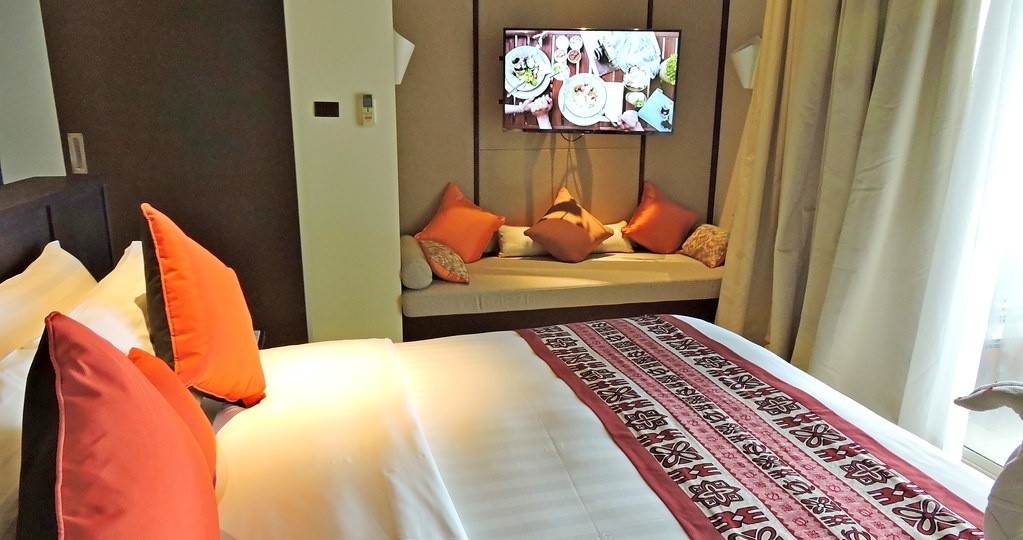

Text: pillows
xmin=400 ymin=235 xmax=432 ymax=290
xmin=497 ymin=219 xmax=634 ymax=257
xmin=0 ymin=240 xmax=98 ymax=359
xmin=621 ymin=180 xmax=701 ymax=254
xmin=15 ymin=311 xmax=221 ymax=540
xmin=139 ymin=202 xmax=267 ymax=409
xmin=523 ymin=187 xmax=615 ymax=263
xmin=418 ymin=239 xmax=470 ymax=285
xmin=128 ymin=347 xmax=217 ymax=484
xmin=414 ymin=182 xmax=505 ymax=263
xmin=679 ymin=224 xmax=730 ymax=269
xmin=0 ymin=240 xmax=157 ymax=540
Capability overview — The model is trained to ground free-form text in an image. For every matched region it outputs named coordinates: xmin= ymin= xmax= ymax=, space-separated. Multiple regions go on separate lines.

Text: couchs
xmin=399 ymin=252 xmax=724 ymax=343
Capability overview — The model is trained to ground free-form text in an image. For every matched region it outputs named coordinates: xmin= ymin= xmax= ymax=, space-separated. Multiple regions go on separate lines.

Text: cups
xmin=625 ymin=91 xmax=646 ymax=107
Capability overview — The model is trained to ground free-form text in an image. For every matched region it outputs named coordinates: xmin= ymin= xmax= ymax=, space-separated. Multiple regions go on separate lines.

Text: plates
xmin=637 ymin=90 xmax=674 ymax=132
xmin=556 ymin=35 xmax=569 ymax=49
xmin=505 ymin=45 xmax=552 ymax=99
xmin=565 ymin=75 xmax=607 ymax=118
xmin=569 ymin=36 xmax=584 ymax=49
xmin=553 ymin=63 xmax=570 ymax=81
xmin=567 ymin=50 xmax=581 ymax=63
xmin=558 ymin=78 xmax=608 ymax=126
xmin=554 ymin=49 xmax=568 ymax=63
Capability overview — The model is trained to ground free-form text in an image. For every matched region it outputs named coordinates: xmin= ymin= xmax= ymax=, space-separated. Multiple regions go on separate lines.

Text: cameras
xmin=594 ymin=36 xmax=617 ymax=63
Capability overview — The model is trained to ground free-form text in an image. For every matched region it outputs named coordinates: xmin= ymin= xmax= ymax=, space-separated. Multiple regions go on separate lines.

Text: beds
xmin=0 ymin=174 xmax=996 ymax=540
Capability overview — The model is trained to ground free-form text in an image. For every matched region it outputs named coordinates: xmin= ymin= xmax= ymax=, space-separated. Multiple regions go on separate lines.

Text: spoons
xmin=600 ymin=110 xmax=617 ymax=127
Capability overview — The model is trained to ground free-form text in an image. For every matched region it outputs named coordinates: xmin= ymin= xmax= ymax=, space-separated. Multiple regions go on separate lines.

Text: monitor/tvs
xmin=503 ymin=27 xmax=682 ymax=136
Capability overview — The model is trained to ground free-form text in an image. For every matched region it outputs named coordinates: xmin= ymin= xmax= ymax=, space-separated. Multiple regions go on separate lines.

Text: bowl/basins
xmin=505 ymin=50 xmax=546 ymax=90
